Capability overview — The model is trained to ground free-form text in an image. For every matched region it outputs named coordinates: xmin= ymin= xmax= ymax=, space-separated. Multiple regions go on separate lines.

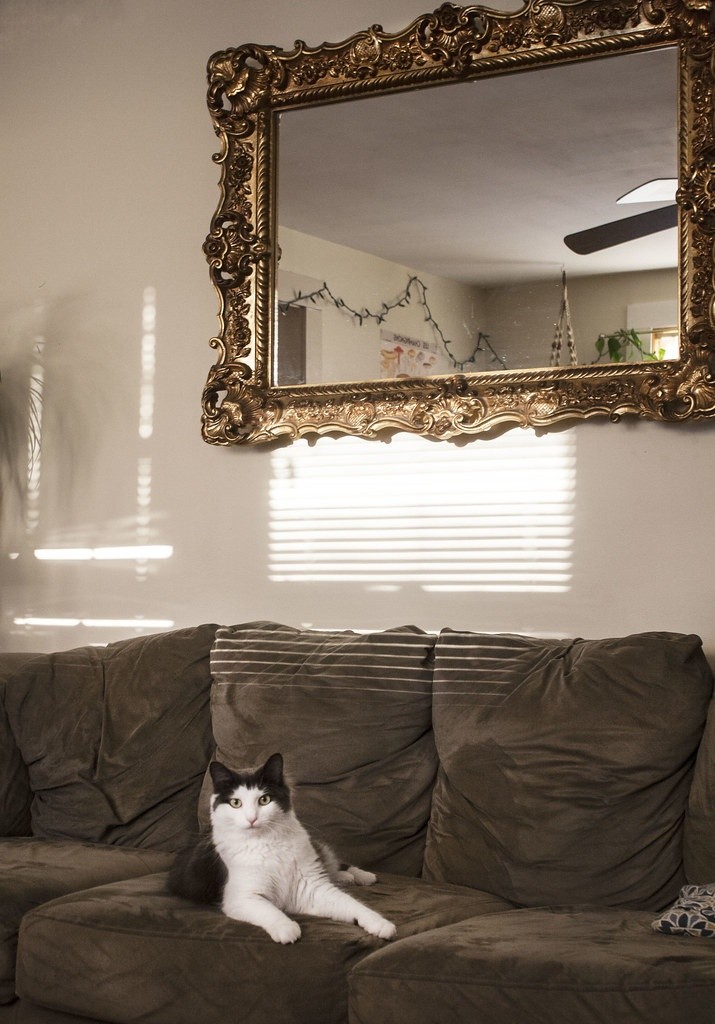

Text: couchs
xmin=0 ymin=620 xmax=715 ymax=1024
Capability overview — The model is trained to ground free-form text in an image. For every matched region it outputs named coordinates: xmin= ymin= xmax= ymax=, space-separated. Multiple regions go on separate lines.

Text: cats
xmin=166 ymin=753 xmax=399 ymax=945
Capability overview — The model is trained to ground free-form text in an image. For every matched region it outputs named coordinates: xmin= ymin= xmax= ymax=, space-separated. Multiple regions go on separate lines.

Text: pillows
xmin=652 ymin=881 xmax=715 ymax=939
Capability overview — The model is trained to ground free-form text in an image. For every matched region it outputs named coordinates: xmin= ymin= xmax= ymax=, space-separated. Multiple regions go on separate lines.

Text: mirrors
xmin=200 ymin=0 xmax=714 ymax=447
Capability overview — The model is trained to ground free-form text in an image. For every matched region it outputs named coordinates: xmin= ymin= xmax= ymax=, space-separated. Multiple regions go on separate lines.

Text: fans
xmin=563 ymin=178 xmax=678 ymax=255
